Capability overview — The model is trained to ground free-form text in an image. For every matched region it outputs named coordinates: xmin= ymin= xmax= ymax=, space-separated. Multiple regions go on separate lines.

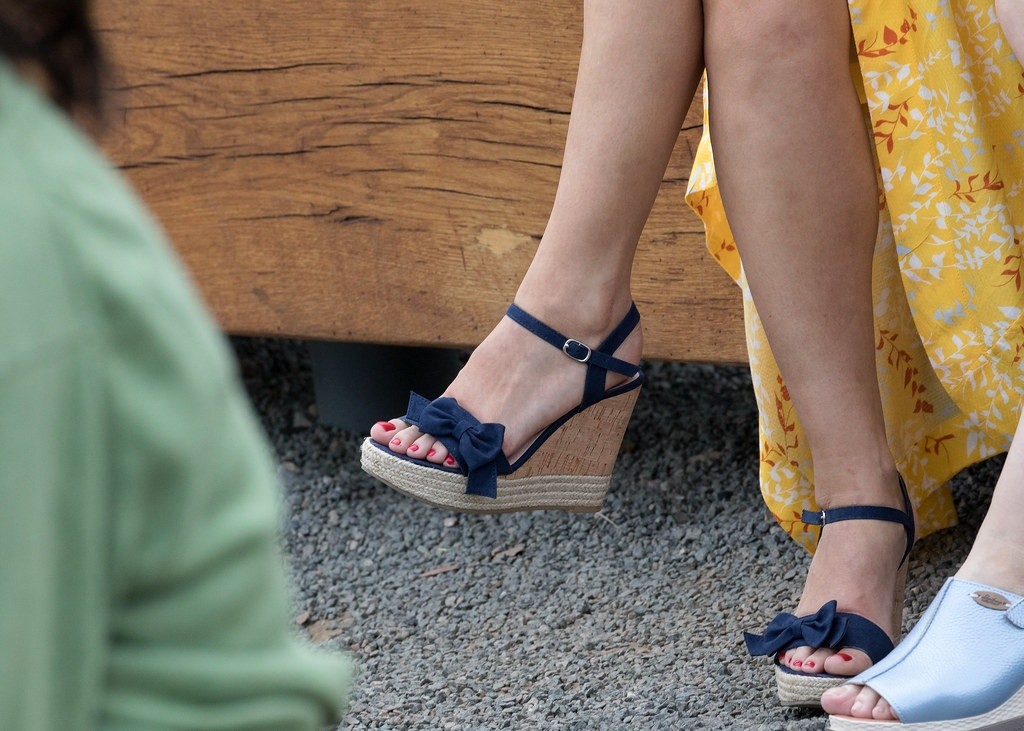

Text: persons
xmin=361 ymin=0 xmax=1024 ymax=705
xmin=820 ymin=410 xmax=1024 ymax=731
xmin=0 ymin=0 xmax=349 ymax=731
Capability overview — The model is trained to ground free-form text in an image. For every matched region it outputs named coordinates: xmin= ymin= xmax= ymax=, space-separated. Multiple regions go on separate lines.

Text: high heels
xmin=743 ymin=471 xmax=916 ymax=706
xmin=360 ymin=300 xmax=645 ymax=513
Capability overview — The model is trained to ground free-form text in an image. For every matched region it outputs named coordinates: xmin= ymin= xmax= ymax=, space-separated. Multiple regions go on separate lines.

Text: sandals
xmin=828 ymin=576 xmax=1024 ymax=731
